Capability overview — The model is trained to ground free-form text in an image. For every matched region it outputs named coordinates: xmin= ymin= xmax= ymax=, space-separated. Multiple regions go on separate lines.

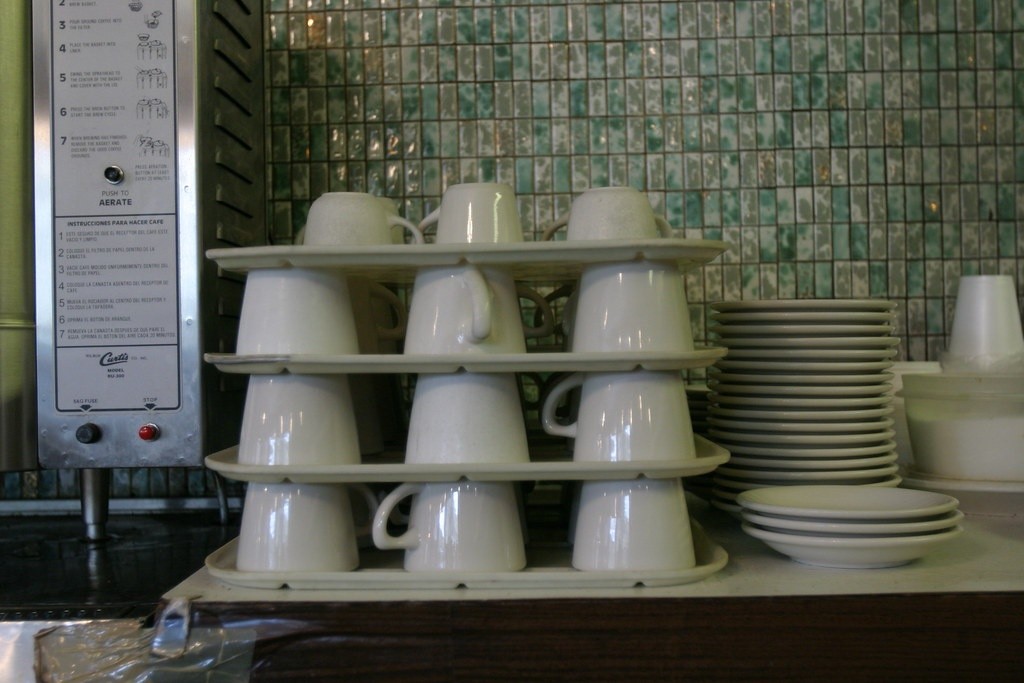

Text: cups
xmin=942 ymin=275 xmax=1024 ymax=369
xmin=374 ymin=481 xmax=530 ymax=573
xmin=541 ymin=371 xmax=697 ymax=462
xmin=411 ymin=182 xmax=522 ymax=246
xmin=234 ymin=483 xmax=363 ymax=572
xmin=303 ymin=192 xmax=423 ymax=245
xmin=235 ymin=374 xmax=362 ymax=467
xmin=402 ymin=266 xmax=556 ymax=355
xmin=235 ymin=268 xmax=407 ymax=356
xmin=571 ymin=478 xmax=697 ymax=571
xmin=535 ymin=264 xmax=693 ymax=353
xmin=403 ymin=372 xmax=531 ymax=465
xmin=540 ymin=185 xmax=674 ymax=242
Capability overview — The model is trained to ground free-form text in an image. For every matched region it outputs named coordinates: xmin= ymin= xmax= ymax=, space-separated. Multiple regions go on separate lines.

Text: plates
xmin=734 ymin=484 xmax=964 ymax=568
xmin=206 ymin=238 xmax=729 ymax=286
xmin=697 ymin=298 xmax=903 ymax=520
xmin=203 ymin=433 xmax=730 ymax=484
xmin=203 ymin=347 xmax=730 ymax=371
xmin=204 ymin=523 xmax=728 ymax=590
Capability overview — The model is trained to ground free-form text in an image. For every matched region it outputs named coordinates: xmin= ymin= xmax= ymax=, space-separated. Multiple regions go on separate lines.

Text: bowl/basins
xmin=895 ymin=373 xmax=1024 ymax=482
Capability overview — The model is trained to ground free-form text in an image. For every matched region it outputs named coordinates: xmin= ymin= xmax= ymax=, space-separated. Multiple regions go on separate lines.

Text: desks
xmin=159 ymin=515 xmax=1024 ymax=683
